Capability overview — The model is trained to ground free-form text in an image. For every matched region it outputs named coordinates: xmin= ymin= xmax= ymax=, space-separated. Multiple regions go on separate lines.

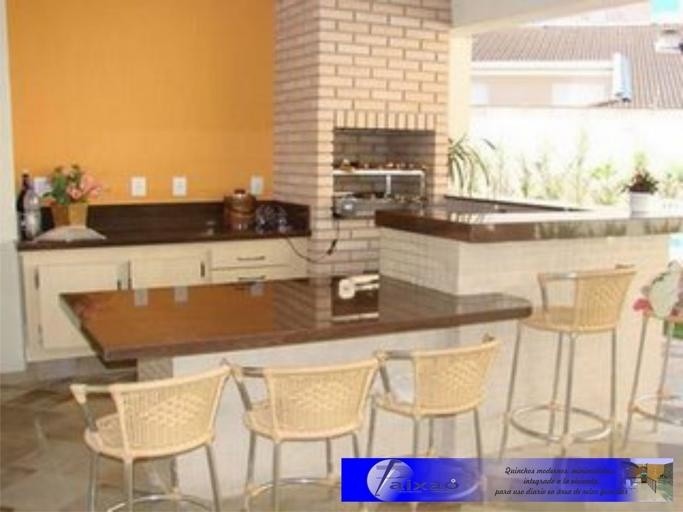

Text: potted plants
xmin=620 ymin=171 xmax=660 ymax=212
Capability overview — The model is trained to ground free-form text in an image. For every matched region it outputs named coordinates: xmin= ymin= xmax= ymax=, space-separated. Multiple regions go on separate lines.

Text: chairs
xmin=624 ymin=307 xmax=683 ymax=439
xmin=69 ymin=357 xmax=231 ymax=512
xmin=221 ymin=351 xmax=386 ymax=512
xmin=367 ymin=332 xmax=499 ymax=511
xmin=500 ymin=263 xmax=637 ymax=459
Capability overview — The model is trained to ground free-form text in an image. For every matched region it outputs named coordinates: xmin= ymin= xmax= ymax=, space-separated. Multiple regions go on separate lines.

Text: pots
xmin=222 ymin=187 xmax=258 ymax=234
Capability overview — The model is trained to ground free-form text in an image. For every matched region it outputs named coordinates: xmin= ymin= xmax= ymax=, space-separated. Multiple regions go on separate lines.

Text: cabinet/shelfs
xmin=210 ymin=238 xmax=306 ymax=284
xmin=25 ymin=248 xmax=206 ymax=363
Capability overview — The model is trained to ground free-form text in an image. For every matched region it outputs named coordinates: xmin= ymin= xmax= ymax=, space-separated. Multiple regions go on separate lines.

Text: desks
xmin=58 ymin=270 xmax=532 ymax=491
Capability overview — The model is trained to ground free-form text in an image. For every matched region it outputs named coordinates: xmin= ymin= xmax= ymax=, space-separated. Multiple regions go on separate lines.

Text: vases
xmin=52 ymin=202 xmax=87 ymax=226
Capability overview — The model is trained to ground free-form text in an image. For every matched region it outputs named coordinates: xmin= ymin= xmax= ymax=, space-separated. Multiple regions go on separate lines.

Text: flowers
xmin=40 ymin=162 xmax=104 ymax=206
